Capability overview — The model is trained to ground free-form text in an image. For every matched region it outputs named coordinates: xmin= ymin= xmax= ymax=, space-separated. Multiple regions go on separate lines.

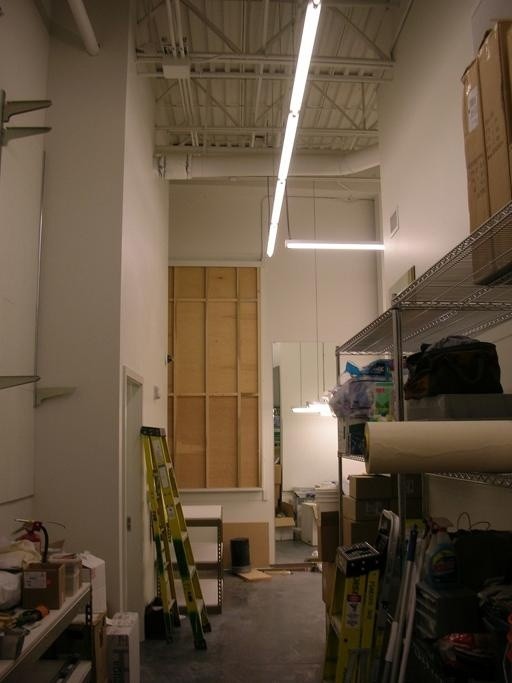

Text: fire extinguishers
xmin=10 ymin=518 xmax=65 ymax=568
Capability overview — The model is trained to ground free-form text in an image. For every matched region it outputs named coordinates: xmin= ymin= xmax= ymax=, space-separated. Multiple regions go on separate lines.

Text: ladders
xmin=323 ymin=542 xmax=378 ymax=683
xmin=141 ymin=425 xmax=211 ymax=650
xmin=379 ymin=513 xmax=430 ymax=683
xmin=369 ymin=509 xmax=399 ymax=683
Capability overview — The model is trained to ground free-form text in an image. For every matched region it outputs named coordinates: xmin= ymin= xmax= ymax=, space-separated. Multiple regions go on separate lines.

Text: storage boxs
xmin=338 ymin=375 xmax=394 ymax=418
xmin=339 ymin=474 xmax=403 ymax=548
xmin=106 ymin=612 xmax=141 ymax=683
xmin=338 ymin=417 xmax=381 ymax=457
xmin=71 ymin=551 xmax=108 ymax=627
xmin=403 ymin=394 xmax=512 ymax=422
xmin=275 ymin=517 xmax=296 ymax=542
xmin=273 ymin=464 xmax=282 ymax=508
xmin=22 ymin=562 xmax=64 ymax=610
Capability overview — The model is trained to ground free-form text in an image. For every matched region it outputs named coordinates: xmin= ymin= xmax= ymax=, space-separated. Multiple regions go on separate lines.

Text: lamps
xmin=265 ymin=0 xmax=323 ymax=258
xmin=282 ymin=182 xmax=384 ymax=250
xmin=288 ymin=177 xmax=335 ymax=418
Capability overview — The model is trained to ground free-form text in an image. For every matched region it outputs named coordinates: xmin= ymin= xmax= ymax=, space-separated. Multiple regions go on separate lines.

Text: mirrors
xmin=273 ymin=366 xmax=280 ymax=465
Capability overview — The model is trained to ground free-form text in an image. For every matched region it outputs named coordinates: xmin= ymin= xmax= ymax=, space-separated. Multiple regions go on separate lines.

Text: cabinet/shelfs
xmin=335 ymin=309 xmax=399 ymax=561
xmin=391 ymin=202 xmax=512 ymax=680
xmin=1 ymin=580 xmax=92 ymax=683
xmin=155 ymin=506 xmax=226 ymax=614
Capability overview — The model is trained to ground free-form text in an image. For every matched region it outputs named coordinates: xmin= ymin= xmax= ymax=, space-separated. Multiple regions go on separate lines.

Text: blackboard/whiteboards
xmin=0 ymin=1 xmax=53 ymax=505
